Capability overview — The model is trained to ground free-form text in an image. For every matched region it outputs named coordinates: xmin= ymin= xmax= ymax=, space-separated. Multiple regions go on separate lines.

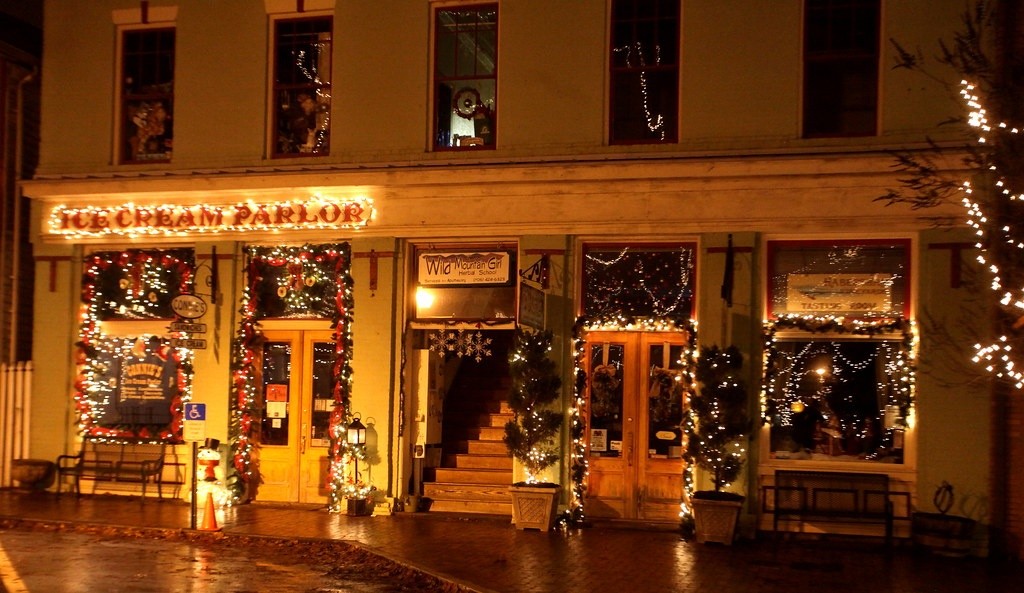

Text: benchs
xmin=771 ymin=469 xmax=895 ymax=549
xmin=54 ymin=433 xmax=169 ymax=507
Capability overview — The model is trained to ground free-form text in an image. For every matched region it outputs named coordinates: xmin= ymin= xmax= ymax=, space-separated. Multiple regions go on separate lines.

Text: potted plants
xmin=499 ymin=323 xmax=566 ymax=534
xmin=681 ymin=340 xmax=755 ymax=547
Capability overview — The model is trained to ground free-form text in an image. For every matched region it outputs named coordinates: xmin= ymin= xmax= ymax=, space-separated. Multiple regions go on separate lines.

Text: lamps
xmin=346 ymin=411 xmax=366 ymax=516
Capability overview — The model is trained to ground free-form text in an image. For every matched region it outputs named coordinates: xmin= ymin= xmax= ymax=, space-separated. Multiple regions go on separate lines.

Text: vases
xmin=402 ymin=495 xmax=417 ymax=513
